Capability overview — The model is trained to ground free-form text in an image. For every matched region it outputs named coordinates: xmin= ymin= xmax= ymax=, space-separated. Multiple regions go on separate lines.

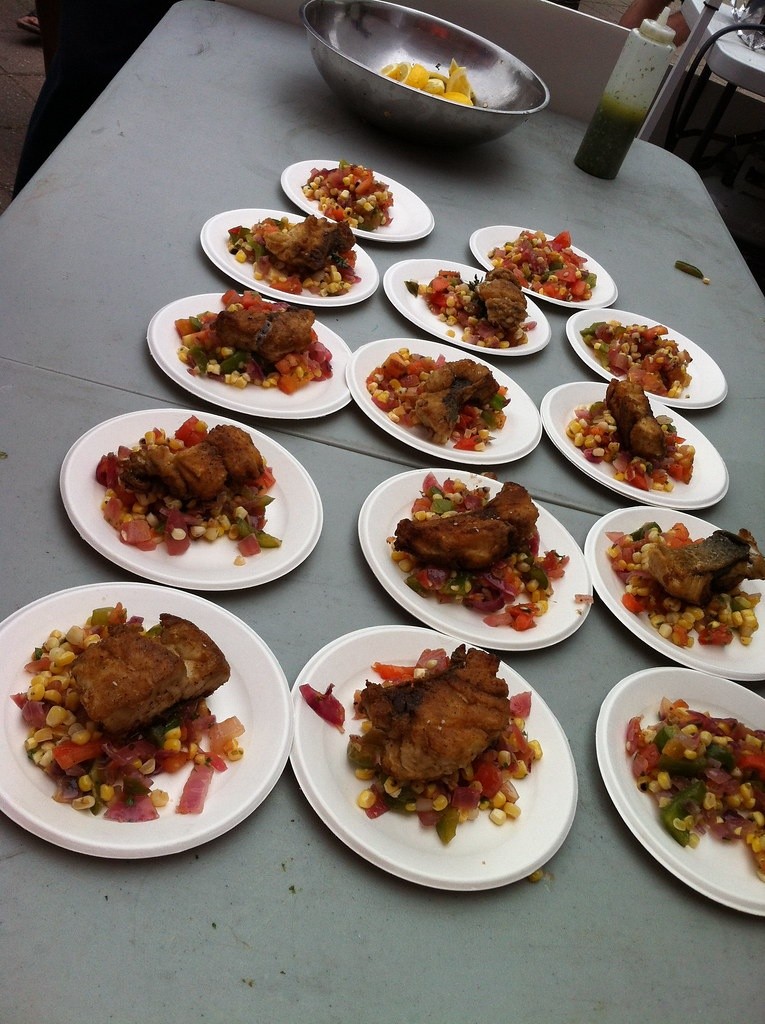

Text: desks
xmin=0 ymin=0 xmax=765 ymax=1024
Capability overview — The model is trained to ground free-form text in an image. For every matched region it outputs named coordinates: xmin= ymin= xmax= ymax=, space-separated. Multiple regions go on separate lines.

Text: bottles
xmin=574 ymin=6 xmax=677 ymax=180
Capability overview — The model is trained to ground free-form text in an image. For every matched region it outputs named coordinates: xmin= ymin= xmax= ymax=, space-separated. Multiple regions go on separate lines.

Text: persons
xmin=618 ymin=0 xmax=691 ymax=47
xmin=13 ymin=0 xmax=172 ymax=196
xmin=17 ymin=0 xmax=42 ymax=36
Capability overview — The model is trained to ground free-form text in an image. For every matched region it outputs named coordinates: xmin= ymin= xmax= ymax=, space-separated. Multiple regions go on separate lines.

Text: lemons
xmin=379 ymin=56 xmax=475 ymax=107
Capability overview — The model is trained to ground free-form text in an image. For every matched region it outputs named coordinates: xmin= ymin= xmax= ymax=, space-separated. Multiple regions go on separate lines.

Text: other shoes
xmin=17 ymin=5 xmax=39 ymax=33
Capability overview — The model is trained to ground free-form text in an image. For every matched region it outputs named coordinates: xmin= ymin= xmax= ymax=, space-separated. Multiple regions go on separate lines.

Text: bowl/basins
xmin=299 ymin=0 xmax=551 ymax=146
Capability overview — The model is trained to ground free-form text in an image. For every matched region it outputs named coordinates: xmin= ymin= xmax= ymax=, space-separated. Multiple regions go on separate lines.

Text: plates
xmin=289 ymin=625 xmax=579 ymax=892
xmin=581 ymin=508 xmax=765 ymax=684
xmin=564 ymin=308 xmax=728 ymax=410
xmin=383 ymin=258 xmax=552 ymax=357
xmin=539 ymin=380 xmax=731 ymax=508
xmin=200 ymin=208 xmax=381 ymax=308
xmin=0 ymin=580 xmax=298 ymax=860
xmin=280 ymin=159 xmax=435 ymax=242
xmin=58 ymin=407 xmax=324 ymax=590
xmin=357 ymin=468 xmax=593 ymax=651
xmin=595 ymin=668 xmax=765 ymax=918
xmin=145 ymin=293 xmax=353 ymax=420
xmin=345 ymin=338 xmax=543 ymax=466
xmin=470 ymin=224 xmax=618 ymax=310
